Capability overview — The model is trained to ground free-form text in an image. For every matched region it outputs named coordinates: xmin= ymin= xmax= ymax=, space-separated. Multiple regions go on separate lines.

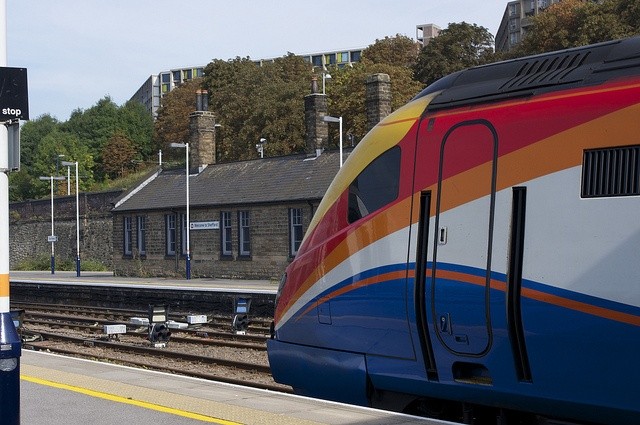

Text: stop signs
xmin=0 ymin=67 xmax=29 ymax=123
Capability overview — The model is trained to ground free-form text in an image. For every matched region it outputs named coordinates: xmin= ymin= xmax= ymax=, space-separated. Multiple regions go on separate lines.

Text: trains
xmin=266 ymin=34 xmax=640 ymax=424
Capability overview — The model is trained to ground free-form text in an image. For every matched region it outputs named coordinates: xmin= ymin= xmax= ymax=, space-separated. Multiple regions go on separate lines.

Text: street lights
xmin=168 ymin=143 xmax=191 ymax=280
xmin=60 ymin=161 xmax=80 ymax=277
xmin=39 ymin=175 xmax=66 ymax=275
xmin=323 ymin=116 xmax=343 ymax=169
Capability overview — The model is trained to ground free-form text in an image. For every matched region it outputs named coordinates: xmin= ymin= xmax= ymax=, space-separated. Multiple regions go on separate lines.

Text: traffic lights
xmin=232 ymin=295 xmax=253 ymax=333
xmin=147 ymin=302 xmax=171 ymax=348
